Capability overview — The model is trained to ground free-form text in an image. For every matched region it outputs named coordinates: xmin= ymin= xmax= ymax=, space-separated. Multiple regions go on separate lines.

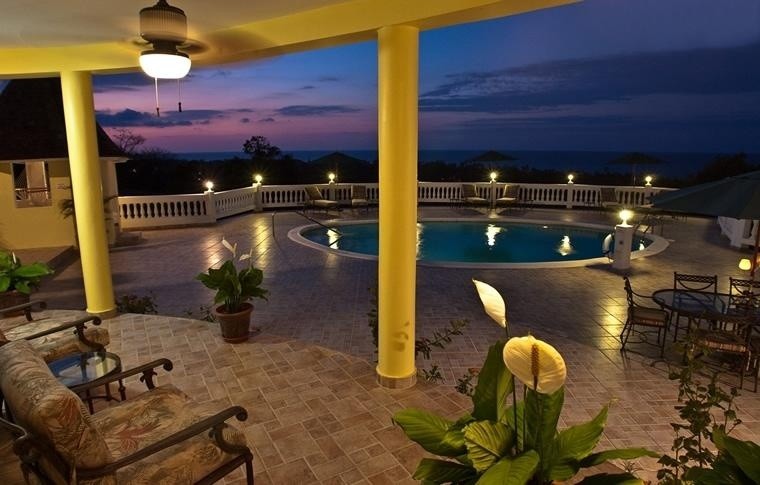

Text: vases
xmin=0 ymin=290 xmax=31 ymax=316
xmin=214 ymin=303 xmax=254 ymax=343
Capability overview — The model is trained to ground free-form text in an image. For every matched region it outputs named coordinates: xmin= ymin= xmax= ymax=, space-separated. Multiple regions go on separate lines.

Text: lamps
xmin=139 ymin=0 xmax=191 ymax=116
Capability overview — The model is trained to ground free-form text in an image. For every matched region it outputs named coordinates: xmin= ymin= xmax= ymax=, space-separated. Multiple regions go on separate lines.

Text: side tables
xmin=47 ymin=350 xmax=125 ymax=412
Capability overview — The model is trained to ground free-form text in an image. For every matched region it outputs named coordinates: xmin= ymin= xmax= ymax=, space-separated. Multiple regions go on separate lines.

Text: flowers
xmin=192 ymin=238 xmax=269 ymax=311
xmin=391 ymin=278 xmax=661 ymax=485
xmin=0 ymin=250 xmax=54 ymax=295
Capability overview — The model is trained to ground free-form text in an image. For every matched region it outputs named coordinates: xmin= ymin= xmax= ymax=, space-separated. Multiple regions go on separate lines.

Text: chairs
xmin=306 ymin=184 xmax=339 ymax=218
xmin=617 ymin=270 xmax=760 ymax=393
xmin=495 ymin=185 xmax=521 ymax=215
xmin=350 ymin=185 xmax=368 ymax=216
xmin=0 ymin=338 xmax=255 ymax=485
xmin=461 ymin=184 xmax=488 ymax=215
xmin=600 ymin=187 xmax=622 ymax=218
xmin=0 ymin=300 xmax=111 ymax=349
